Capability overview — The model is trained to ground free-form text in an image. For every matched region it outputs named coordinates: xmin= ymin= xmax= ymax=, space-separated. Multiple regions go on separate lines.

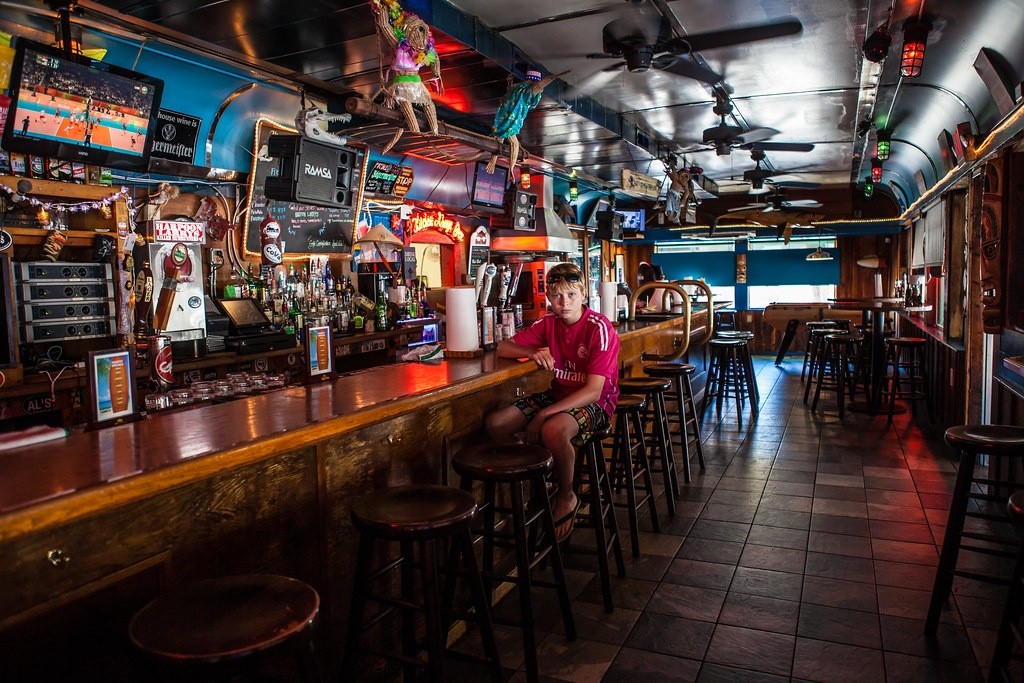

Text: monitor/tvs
xmin=470 ymin=160 xmax=509 ymax=208
xmin=215 ymin=297 xmax=272 ymax=329
xmin=613 ymin=209 xmax=645 ymax=233
xmin=1 ymin=37 xmax=164 ymax=173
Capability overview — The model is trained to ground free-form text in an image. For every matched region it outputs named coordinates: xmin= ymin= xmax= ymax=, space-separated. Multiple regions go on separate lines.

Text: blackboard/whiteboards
xmin=243 ymin=118 xmax=369 ymax=259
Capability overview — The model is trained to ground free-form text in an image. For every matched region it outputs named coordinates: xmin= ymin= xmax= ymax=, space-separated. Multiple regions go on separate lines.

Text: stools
xmin=118 ymin=308 xmax=1024 ymax=683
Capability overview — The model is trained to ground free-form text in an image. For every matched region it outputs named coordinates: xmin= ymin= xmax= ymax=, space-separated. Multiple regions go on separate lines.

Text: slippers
xmin=553 ymin=498 xmax=581 ymax=543
xmin=544 ymin=479 xmax=558 ymax=498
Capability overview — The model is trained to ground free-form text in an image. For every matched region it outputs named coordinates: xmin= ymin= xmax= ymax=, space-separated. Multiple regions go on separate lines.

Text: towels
xmin=0 ymin=424 xmax=68 ymax=452
xmin=399 ymin=344 xmax=445 ymax=362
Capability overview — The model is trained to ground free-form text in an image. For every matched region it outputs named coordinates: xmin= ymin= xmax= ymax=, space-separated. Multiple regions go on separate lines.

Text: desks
xmin=828 ymin=302 xmax=932 ymax=418
xmin=826 ymin=295 xmax=906 ymax=386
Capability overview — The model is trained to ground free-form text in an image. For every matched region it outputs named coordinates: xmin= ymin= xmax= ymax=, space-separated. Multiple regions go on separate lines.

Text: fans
xmin=526 ymin=0 xmax=804 ymax=102
xmin=671 ymin=104 xmax=815 ymax=157
xmin=727 ymin=189 xmax=822 ymax=212
xmin=710 ymin=153 xmax=819 ymax=194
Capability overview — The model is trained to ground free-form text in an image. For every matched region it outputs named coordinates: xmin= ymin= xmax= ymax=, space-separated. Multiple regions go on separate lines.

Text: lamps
xmin=863 ymin=176 xmax=875 ymax=197
xmin=870 ymin=157 xmax=885 ymax=184
xmin=896 ymin=14 xmax=934 ymax=80
xmin=876 ymin=128 xmax=894 ymax=161
xmin=805 ymin=225 xmax=834 ymax=261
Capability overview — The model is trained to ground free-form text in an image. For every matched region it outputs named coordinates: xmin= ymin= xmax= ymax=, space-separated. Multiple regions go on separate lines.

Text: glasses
xmin=547 ymin=271 xmax=584 ymax=285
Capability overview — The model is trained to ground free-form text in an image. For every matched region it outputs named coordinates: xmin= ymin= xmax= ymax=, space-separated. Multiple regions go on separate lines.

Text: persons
xmin=487 ymin=263 xmax=620 ymax=543
xmin=22 ymin=62 xmax=150 ymax=147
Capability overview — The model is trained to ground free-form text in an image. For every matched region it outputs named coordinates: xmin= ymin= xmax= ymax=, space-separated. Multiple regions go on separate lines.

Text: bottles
xmin=375 ymin=293 xmax=387 ymax=331
xmin=352 ymin=308 xmax=365 ymax=331
xmin=351 ymin=291 xmax=375 ymax=314
xmin=326 ymin=261 xmax=335 ymax=296
xmin=335 ymin=304 xmax=349 ymax=332
xmin=238 ymin=269 xmax=249 ymax=299
xmin=247 ymin=263 xmax=257 ymax=298
xmin=264 ymin=258 xmax=329 ymax=347
xmin=259 ymin=273 xmax=270 ymax=305
xmin=223 ymin=265 xmax=241 ymax=298
xmin=406 ymin=297 xmax=423 ymax=320
xmin=336 ymin=275 xmax=355 ymax=303
xmin=365 ymin=310 xmax=375 ymax=333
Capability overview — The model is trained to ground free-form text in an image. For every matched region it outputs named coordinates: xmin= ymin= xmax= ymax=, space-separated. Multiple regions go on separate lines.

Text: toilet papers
xmin=599 ymin=281 xmax=618 ymax=323
xmin=445 ymin=288 xmax=480 ymax=351
xmin=874 ymin=274 xmax=883 ymax=308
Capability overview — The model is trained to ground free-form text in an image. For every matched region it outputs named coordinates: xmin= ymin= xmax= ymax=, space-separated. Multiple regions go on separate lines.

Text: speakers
xmin=510 ymin=190 xmax=538 ymax=230
xmin=594 ymin=211 xmax=625 ymax=242
xmin=264 ymin=133 xmax=358 ymax=210
